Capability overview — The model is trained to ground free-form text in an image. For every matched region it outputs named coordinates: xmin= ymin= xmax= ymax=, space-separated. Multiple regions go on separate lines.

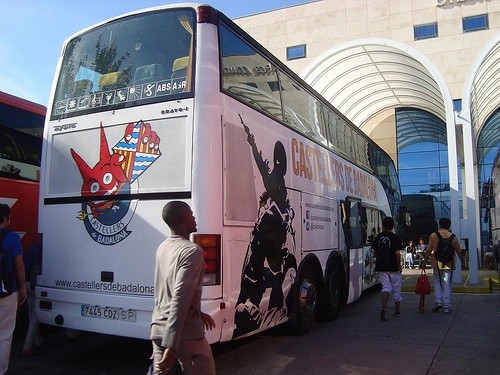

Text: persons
xmin=485 ymin=240 xmax=500 ymax=270
xmin=150 ymin=201 xmax=217 ymax=375
xmin=406 ymin=238 xmax=428 ymax=271
xmin=420 ymin=217 xmax=465 ymax=313
xmin=373 ymin=217 xmax=403 ymax=320
xmin=24 ymin=244 xmax=47 ymax=357
xmin=0 ymin=204 xmax=27 ymax=375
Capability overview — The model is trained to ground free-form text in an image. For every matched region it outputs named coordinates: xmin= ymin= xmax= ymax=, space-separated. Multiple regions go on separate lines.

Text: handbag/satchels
xmin=414 ymin=269 xmax=430 ymax=295
xmin=419 ymin=295 xmax=425 ymax=311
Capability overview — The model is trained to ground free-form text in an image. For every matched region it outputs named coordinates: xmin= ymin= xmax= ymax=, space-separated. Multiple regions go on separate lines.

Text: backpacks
xmin=434 ymin=230 xmax=456 ymax=264
xmin=0 ymin=228 xmax=12 ymax=298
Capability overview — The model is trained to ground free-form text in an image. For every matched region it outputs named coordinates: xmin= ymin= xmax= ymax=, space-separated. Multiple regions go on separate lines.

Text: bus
xmin=0 ymin=90 xmax=48 ymax=280
xmin=34 ymin=1 xmax=411 ymax=348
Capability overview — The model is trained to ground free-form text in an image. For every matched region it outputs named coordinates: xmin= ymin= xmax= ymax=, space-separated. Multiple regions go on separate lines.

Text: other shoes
xmin=444 ymin=308 xmax=450 ymax=312
xmin=409 ymin=267 xmax=413 ymax=270
xmin=432 ymin=304 xmax=443 ymax=311
xmin=415 ymin=266 xmax=420 ymax=270
xmin=411 ymin=264 xmax=413 ymax=266
xmin=22 ymin=350 xmax=41 ymax=357
xmin=381 ymin=308 xmax=387 ymax=321
xmin=395 ymin=312 xmax=400 ymax=317
xmin=36 ymin=343 xmax=47 ymax=348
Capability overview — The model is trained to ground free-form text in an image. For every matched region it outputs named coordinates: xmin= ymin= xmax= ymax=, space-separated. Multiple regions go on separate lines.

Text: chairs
xmin=173 ymin=57 xmax=190 ymax=87
xmin=99 ymin=71 xmax=126 ymax=92
xmin=69 ymin=79 xmax=93 ymax=96
xmin=134 ymin=64 xmax=164 ymax=84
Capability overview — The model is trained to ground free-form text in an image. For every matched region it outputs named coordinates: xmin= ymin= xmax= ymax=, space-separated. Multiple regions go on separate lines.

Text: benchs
xmin=403 ymin=252 xmax=431 ymax=270
